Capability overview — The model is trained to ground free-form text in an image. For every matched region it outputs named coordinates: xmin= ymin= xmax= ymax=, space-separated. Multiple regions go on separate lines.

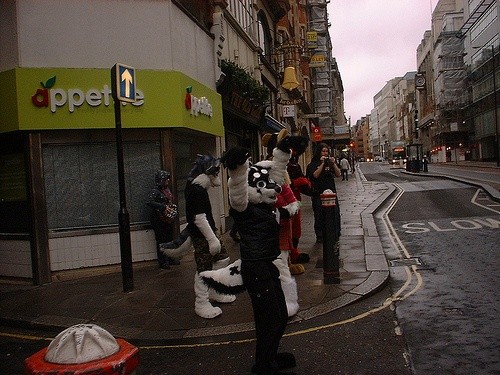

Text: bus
xmin=388 ymin=141 xmax=407 ymax=165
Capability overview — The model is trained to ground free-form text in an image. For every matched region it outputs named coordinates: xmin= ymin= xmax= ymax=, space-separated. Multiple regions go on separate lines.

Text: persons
xmin=340 ymin=156 xmax=349 ymax=181
xmin=306 ymin=143 xmax=341 ymax=244
xmin=145 ymin=169 xmax=181 ymax=271
xmin=336 ymin=153 xmax=361 ymax=172
xmin=328 ymin=153 xmax=336 ymax=163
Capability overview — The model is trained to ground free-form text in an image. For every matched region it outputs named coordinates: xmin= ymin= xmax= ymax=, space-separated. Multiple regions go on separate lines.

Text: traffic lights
xmin=350 ymin=143 xmax=355 ymax=148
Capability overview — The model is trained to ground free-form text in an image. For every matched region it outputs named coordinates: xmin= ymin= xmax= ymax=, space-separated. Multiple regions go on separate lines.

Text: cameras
xmin=324 ymin=156 xmax=333 ymax=164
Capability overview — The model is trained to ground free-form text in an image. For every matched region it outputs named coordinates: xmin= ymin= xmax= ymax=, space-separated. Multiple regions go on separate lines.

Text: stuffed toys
xmin=198 ymin=134 xmax=296 ymax=375
xmin=158 ymin=153 xmax=237 ymax=318
xmin=259 ymin=132 xmax=322 ymax=277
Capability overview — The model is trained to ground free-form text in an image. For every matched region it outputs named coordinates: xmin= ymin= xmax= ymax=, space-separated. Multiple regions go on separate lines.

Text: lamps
xmin=258 ymin=38 xmax=299 ymax=91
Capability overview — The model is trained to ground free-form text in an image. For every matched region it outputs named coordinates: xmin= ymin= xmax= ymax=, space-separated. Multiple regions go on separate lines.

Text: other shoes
xmin=346 ymin=178 xmax=348 ymax=181
xmin=342 ymin=179 xmax=345 ymax=181
